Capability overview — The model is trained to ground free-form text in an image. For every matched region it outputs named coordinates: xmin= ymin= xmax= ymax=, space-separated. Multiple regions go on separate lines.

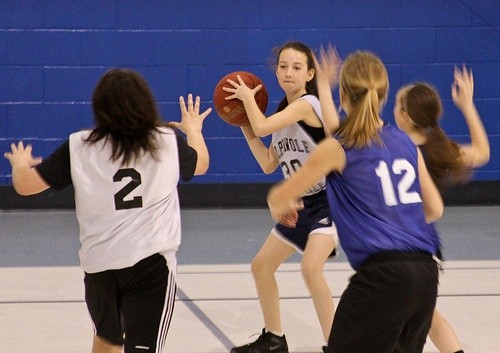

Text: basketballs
xmin=213 ymin=71 xmax=269 ymax=127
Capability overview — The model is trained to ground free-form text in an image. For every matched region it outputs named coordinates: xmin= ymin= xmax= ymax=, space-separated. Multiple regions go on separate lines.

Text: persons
xmin=222 ymin=43 xmax=339 ymax=353
xmin=394 ymin=62 xmax=491 ymax=353
xmin=5 ymin=67 xmax=213 ymax=353
xmin=268 ymin=51 xmax=445 ymax=353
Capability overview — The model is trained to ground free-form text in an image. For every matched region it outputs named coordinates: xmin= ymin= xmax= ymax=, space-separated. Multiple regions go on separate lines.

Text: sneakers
xmin=230 ymin=331 xmax=288 ymax=353
